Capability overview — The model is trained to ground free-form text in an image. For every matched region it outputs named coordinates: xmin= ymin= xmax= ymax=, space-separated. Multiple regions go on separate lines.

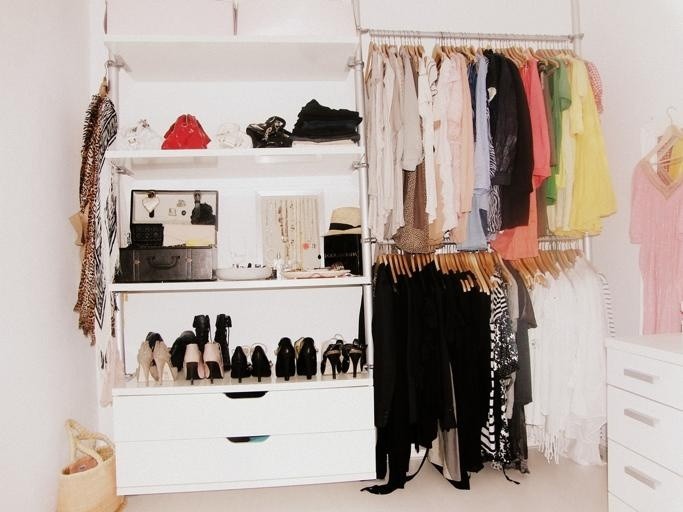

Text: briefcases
xmin=120 ymin=190 xmax=218 ymax=282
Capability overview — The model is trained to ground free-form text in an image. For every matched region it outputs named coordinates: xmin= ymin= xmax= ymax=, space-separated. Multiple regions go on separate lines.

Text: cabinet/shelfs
xmin=599 ymin=332 xmax=683 ymax=512
xmin=89 ymin=0 xmax=380 ymax=497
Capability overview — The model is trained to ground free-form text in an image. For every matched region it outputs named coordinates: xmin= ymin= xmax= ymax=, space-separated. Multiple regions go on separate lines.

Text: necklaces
xmin=263 ymin=196 xmax=322 ymax=263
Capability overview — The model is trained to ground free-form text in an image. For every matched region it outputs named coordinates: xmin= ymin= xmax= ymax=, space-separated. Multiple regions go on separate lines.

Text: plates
xmin=215 ymin=267 xmax=273 ymax=281
xmin=280 ymin=269 xmax=351 ymax=279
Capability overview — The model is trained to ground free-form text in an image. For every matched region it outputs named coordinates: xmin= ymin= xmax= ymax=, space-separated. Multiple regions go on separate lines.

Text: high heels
xmin=136 ymin=314 xmax=367 ymax=386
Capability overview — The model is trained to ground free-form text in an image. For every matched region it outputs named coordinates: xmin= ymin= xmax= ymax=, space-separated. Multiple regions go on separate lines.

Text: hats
xmin=320 ymin=207 xmax=361 ymax=237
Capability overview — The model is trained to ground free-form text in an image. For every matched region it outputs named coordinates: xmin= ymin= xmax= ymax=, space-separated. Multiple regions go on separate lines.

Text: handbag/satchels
xmin=60 ymin=445 xmax=124 ymax=512
xmin=121 ymin=114 xmax=294 ymax=150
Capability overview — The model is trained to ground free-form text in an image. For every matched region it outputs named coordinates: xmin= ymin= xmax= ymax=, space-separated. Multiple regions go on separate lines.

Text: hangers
xmin=359 ymin=28 xmax=583 ymax=87
xmin=637 ymin=103 xmax=683 ymax=172
xmin=94 ymin=56 xmax=114 ymax=96
xmin=364 ymin=238 xmax=587 ymax=300
